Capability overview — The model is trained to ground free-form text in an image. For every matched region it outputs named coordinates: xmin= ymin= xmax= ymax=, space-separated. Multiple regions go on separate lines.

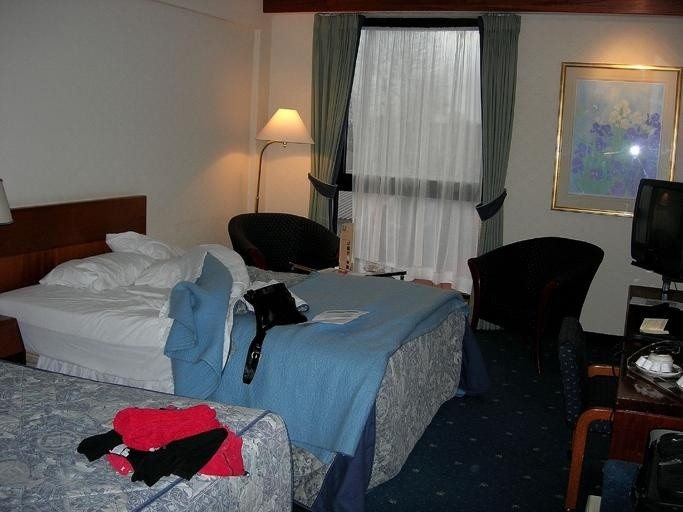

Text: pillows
xmin=35 ymin=250 xmax=153 ymax=294
xmin=100 ymin=226 xmax=190 ymax=261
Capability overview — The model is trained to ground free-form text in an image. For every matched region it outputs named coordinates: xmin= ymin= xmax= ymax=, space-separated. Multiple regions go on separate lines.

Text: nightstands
xmin=0 ymin=310 xmax=26 ymax=368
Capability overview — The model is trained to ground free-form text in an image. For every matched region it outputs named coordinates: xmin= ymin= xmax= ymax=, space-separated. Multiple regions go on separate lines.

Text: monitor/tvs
xmin=630 ymin=178 xmax=683 ymax=283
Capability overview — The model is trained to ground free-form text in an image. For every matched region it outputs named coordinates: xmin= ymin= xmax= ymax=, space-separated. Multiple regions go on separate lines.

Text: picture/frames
xmin=548 ymin=60 xmax=682 ymax=220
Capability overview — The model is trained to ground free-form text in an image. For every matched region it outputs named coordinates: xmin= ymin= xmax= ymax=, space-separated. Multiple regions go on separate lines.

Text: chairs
xmin=463 ymin=236 xmax=607 ymax=376
xmin=563 ymin=406 xmax=647 ymax=512
xmin=557 ymin=313 xmax=620 ymax=458
xmin=227 ymin=211 xmax=339 ymax=276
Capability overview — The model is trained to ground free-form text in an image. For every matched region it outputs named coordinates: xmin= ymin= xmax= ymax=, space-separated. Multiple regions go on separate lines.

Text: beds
xmin=0 ymin=358 xmax=294 ymax=512
xmin=0 ymin=194 xmax=471 ymax=512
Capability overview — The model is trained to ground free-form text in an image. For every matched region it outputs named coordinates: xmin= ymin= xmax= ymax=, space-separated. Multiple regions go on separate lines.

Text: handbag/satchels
xmin=241 ymin=283 xmax=309 ymax=384
xmin=629 ymin=428 xmax=683 ymax=510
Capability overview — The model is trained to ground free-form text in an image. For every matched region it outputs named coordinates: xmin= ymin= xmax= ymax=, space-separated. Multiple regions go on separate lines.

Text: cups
xmin=635 ymin=355 xmax=673 ymax=372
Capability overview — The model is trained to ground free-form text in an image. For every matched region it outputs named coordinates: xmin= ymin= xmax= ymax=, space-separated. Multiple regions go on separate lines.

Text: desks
xmin=608 ymin=282 xmax=682 ymax=463
xmin=284 ymin=256 xmax=408 ymax=281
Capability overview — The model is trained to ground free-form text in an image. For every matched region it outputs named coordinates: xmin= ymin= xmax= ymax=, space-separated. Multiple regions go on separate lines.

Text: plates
xmin=635 ymin=362 xmax=681 ymax=376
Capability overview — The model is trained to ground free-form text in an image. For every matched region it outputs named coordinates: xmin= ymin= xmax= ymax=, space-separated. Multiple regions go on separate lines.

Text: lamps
xmin=0 ymin=177 xmax=16 ymax=227
xmin=253 ymin=108 xmax=315 ymax=213
